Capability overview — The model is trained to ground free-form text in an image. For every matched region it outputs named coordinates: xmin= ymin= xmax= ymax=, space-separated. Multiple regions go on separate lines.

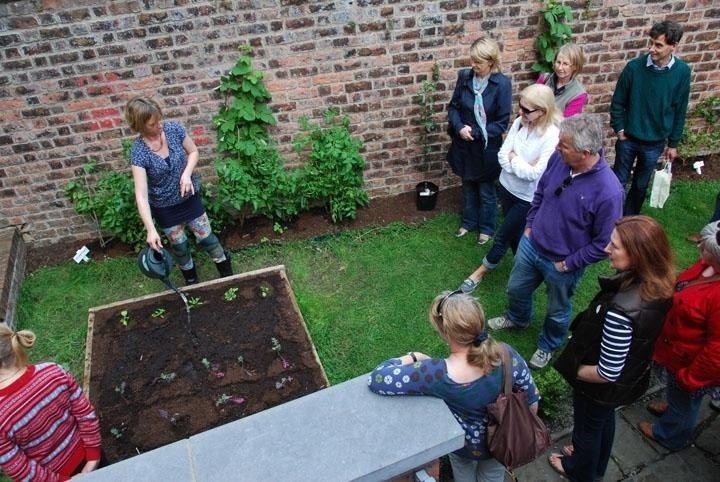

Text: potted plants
xmin=416 ymin=57 xmax=439 ymax=211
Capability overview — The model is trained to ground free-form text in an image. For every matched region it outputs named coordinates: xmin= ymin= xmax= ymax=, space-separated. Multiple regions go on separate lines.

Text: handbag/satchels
xmin=485 ymin=343 xmax=554 ymax=471
xmin=649 ymin=161 xmax=673 ymax=208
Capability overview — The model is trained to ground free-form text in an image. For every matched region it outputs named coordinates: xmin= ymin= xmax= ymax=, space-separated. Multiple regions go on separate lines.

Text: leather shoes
xmin=709 ymin=398 xmax=720 ymax=412
xmin=646 ymin=399 xmax=667 ymax=417
xmin=637 ymin=421 xmax=658 ymax=441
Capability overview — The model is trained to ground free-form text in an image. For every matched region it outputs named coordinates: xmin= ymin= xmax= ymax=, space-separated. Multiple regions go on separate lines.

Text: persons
xmin=368 ymin=289 xmax=541 ymax=482
xmin=609 ymin=21 xmax=691 ymax=218
xmin=446 ymin=36 xmax=512 ymax=245
xmin=0 ymin=324 xmax=102 ymax=482
xmin=547 ymin=215 xmax=676 ymax=482
xmin=125 ymin=96 xmax=232 ymax=286
xmin=488 ymin=116 xmax=625 ymax=371
xmin=686 ymin=201 xmax=716 ymax=246
xmin=456 ymin=83 xmax=564 ymax=296
xmin=520 ymin=42 xmax=587 ymax=117
xmin=639 ymin=221 xmax=720 ymax=452
xmin=516 ymin=78 xmax=519 ymax=81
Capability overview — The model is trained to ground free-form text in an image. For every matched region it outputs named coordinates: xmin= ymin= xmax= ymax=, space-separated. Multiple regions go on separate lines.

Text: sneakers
xmin=528 ymin=348 xmax=557 ymax=370
xmin=485 ymin=315 xmax=530 ymax=331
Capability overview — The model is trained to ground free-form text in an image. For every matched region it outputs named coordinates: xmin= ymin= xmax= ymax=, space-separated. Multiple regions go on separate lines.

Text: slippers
xmin=561 ymin=447 xmax=575 ymax=456
xmin=548 ymin=453 xmax=604 ymax=482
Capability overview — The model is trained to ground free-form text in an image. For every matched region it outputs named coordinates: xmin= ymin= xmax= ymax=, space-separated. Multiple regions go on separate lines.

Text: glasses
xmin=555 ymin=176 xmax=572 ymax=197
xmin=437 ymin=289 xmax=464 ymax=315
xmin=716 ymin=221 xmax=720 ymax=246
xmin=519 ymin=98 xmax=539 ymax=113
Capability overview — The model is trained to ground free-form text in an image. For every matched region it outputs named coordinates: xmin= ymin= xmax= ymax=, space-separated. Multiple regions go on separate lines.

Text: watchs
xmin=563 ymin=261 xmax=569 ymax=272
xmin=407 ymin=352 xmax=418 ymax=363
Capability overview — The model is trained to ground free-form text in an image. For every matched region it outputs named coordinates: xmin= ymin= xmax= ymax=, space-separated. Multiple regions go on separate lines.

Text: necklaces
xmin=146 ymin=133 xmax=164 ymax=152
xmin=0 ymin=367 xmax=21 ymax=384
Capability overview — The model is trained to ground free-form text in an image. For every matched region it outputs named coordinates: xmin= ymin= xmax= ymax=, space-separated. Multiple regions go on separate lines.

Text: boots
xmin=179 ymin=261 xmax=198 ymax=286
xmin=214 ymin=257 xmax=233 ymax=278
